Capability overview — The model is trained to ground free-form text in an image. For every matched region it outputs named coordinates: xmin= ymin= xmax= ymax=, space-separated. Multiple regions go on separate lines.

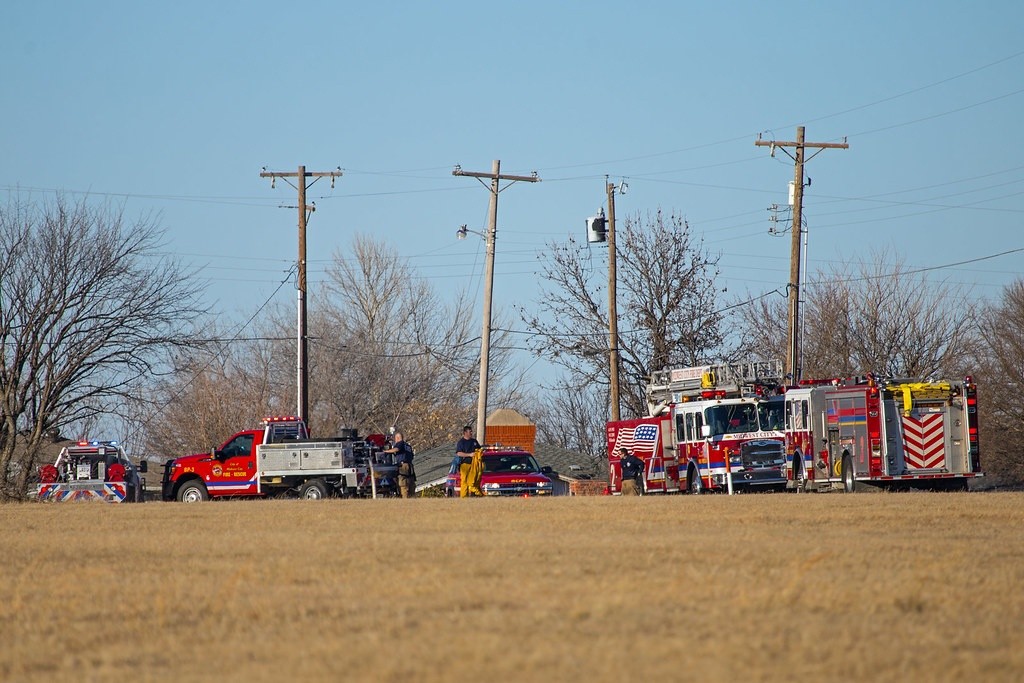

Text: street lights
xmin=454 ymin=224 xmax=496 ymax=446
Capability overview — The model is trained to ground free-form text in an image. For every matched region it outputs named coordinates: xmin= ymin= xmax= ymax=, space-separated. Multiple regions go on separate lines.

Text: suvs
xmin=445 ymin=443 xmax=555 ymax=497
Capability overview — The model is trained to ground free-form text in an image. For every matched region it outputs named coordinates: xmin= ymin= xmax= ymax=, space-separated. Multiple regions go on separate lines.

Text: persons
xmin=456 ymin=426 xmax=481 ymax=498
xmin=383 ymin=433 xmax=414 ymax=498
xmin=618 ymin=448 xmax=645 ymax=494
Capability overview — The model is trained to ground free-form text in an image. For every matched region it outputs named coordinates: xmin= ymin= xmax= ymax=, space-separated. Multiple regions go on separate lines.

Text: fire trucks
xmin=607 ymin=359 xmax=985 ymax=495
xmin=157 ymin=415 xmax=403 ymax=502
xmin=34 ymin=439 xmax=149 ymax=504
